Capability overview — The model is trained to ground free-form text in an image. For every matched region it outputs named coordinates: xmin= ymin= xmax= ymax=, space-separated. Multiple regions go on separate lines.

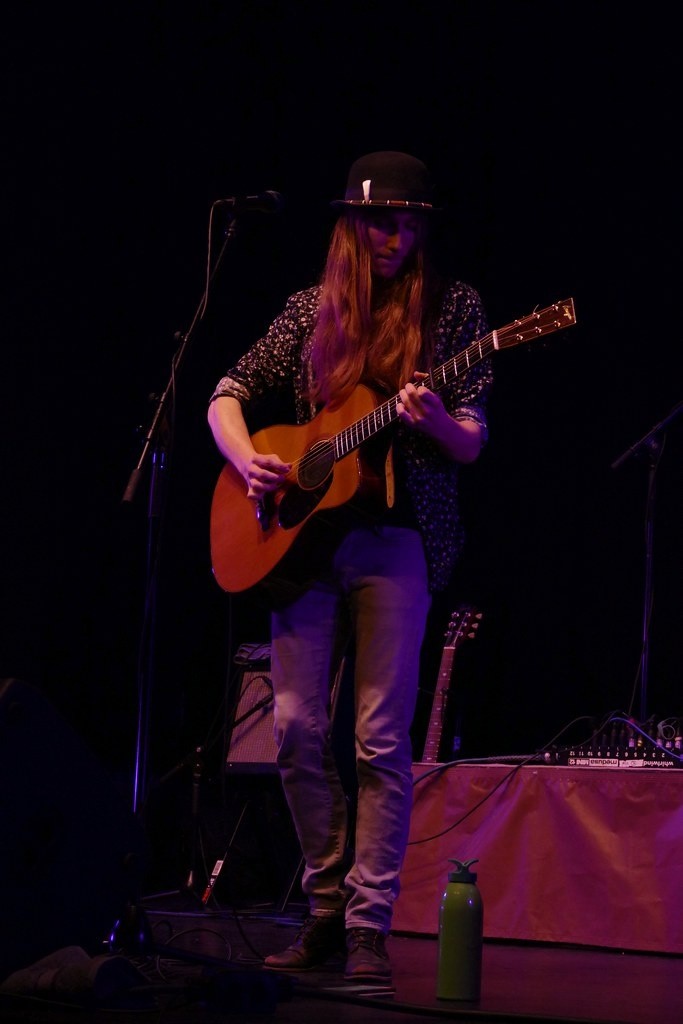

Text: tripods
xmin=98 ymin=215 xmax=241 ymax=977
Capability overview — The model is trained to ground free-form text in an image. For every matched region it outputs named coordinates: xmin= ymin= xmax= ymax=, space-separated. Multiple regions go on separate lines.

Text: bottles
xmin=437 ymin=857 xmax=484 ymax=1001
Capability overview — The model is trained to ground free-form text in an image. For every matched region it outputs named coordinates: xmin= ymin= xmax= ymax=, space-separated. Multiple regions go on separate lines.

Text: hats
xmin=331 ymin=150 xmax=445 ymax=212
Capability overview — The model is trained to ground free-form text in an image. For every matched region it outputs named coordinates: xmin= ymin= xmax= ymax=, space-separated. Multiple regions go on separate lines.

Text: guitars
xmin=418 ymin=607 xmax=481 ymax=763
xmin=207 ymin=297 xmax=578 ymax=608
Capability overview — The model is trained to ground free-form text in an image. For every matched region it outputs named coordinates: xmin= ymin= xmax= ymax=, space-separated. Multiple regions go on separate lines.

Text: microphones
xmin=229 ymin=190 xmax=284 ymax=214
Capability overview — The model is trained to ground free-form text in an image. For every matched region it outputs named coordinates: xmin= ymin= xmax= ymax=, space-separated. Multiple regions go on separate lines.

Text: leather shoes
xmin=342 ymin=927 xmax=393 ymax=982
xmin=260 ymin=915 xmax=347 ymax=972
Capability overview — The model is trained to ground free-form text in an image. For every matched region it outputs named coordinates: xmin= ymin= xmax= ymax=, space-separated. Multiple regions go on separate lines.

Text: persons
xmin=204 ymin=154 xmax=493 ymax=994
